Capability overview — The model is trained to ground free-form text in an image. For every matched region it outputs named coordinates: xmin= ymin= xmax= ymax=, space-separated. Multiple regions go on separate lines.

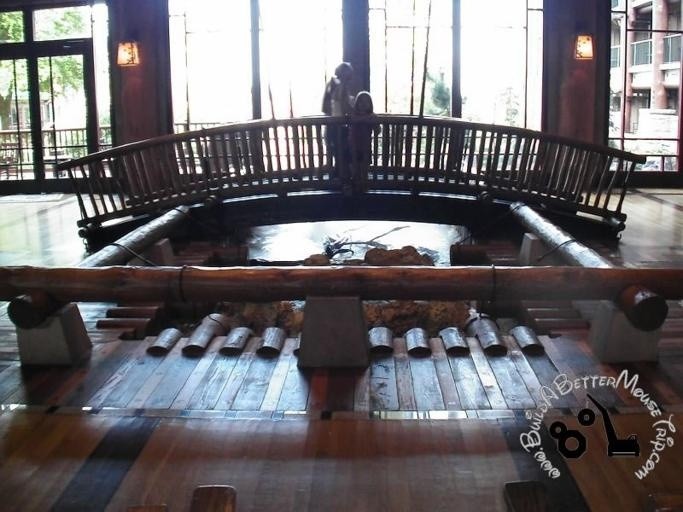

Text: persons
xmin=321 ymin=62 xmax=357 ymax=165
xmin=348 ymin=93 xmax=381 ymax=180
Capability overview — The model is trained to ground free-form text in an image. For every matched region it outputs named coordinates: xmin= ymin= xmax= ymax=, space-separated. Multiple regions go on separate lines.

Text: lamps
xmin=115 ymin=21 xmax=142 ymax=68
xmin=572 ymin=19 xmax=595 ymax=62
